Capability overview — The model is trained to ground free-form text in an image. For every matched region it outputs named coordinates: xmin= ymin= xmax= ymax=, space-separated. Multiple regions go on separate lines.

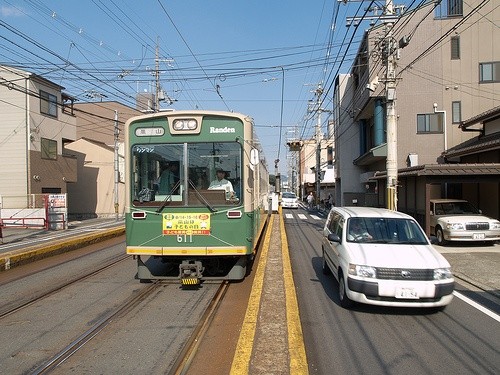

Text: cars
xmin=321 ymin=207 xmax=454 ymax=312
xmin=282 ymin=192 xmax=299 ymax=209
xmin=429 ymin=199 xmax=500 ymax=245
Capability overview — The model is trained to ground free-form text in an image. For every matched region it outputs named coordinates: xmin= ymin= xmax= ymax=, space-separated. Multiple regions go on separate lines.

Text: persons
xmin=348 ymin=219 xmax=374 ymax=241
xmin=207 ymin=168 xmax=236 ymax=201
xmin=306 ymin=191 xmax=315 ymax=210
xmin=326 ymin=192 xmax=334 ymax=210
xmin=158 ymin=161 xmax=179 ymax=195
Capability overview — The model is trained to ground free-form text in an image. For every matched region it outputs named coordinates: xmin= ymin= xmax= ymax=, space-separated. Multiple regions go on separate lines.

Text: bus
xmin=123 ymin=111 xmax=268 ymax=285
